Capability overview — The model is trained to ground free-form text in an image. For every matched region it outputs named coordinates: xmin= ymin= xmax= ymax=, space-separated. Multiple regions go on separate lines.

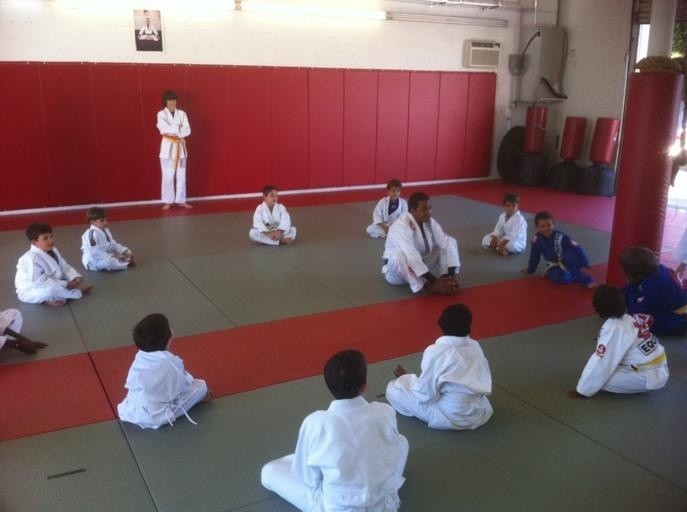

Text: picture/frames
xmin=132 ymin=8 xmax=165 ymax=53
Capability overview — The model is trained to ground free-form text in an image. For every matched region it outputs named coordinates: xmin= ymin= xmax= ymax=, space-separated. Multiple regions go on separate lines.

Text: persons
xmin=483 ymin=192 xmax=527 ymax=256
xmin=117 ymin=312 xmax=215 ymax=430
xmin=249 ymin=183 xmax=297 ymax=246
xmin=138 ymin=18 xmax=159 ymax=42
xmin=366 ymin=179 xmax=408 ymax=237
xmin=157 ymin=89 xmax=193 ymax=211
xmin=521 ymin=210 xmax=599 ymax=289
xmin=385 ymin=302 xmax=494 ymax=430
xmin=380 ymin=190 xmax=461 ymax=295
xmin=568 ymin=283 xmax=669 ymax=398
xmin=79 ymin=206 xmax=136 ymax=271
xmin=621 ymin=245 xmax=687 ymax=336
xmin=674 ymin=230 xmax=686 ymax=279
xmin=1 ymin=222 xmax=92 ymax=355
xmin=260 ymin=349 xmax=409 ymax=511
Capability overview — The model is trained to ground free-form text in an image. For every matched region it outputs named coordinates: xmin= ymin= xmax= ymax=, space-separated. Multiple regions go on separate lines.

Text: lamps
xmin=385 ymin=10 xmax=508 ymax=29
